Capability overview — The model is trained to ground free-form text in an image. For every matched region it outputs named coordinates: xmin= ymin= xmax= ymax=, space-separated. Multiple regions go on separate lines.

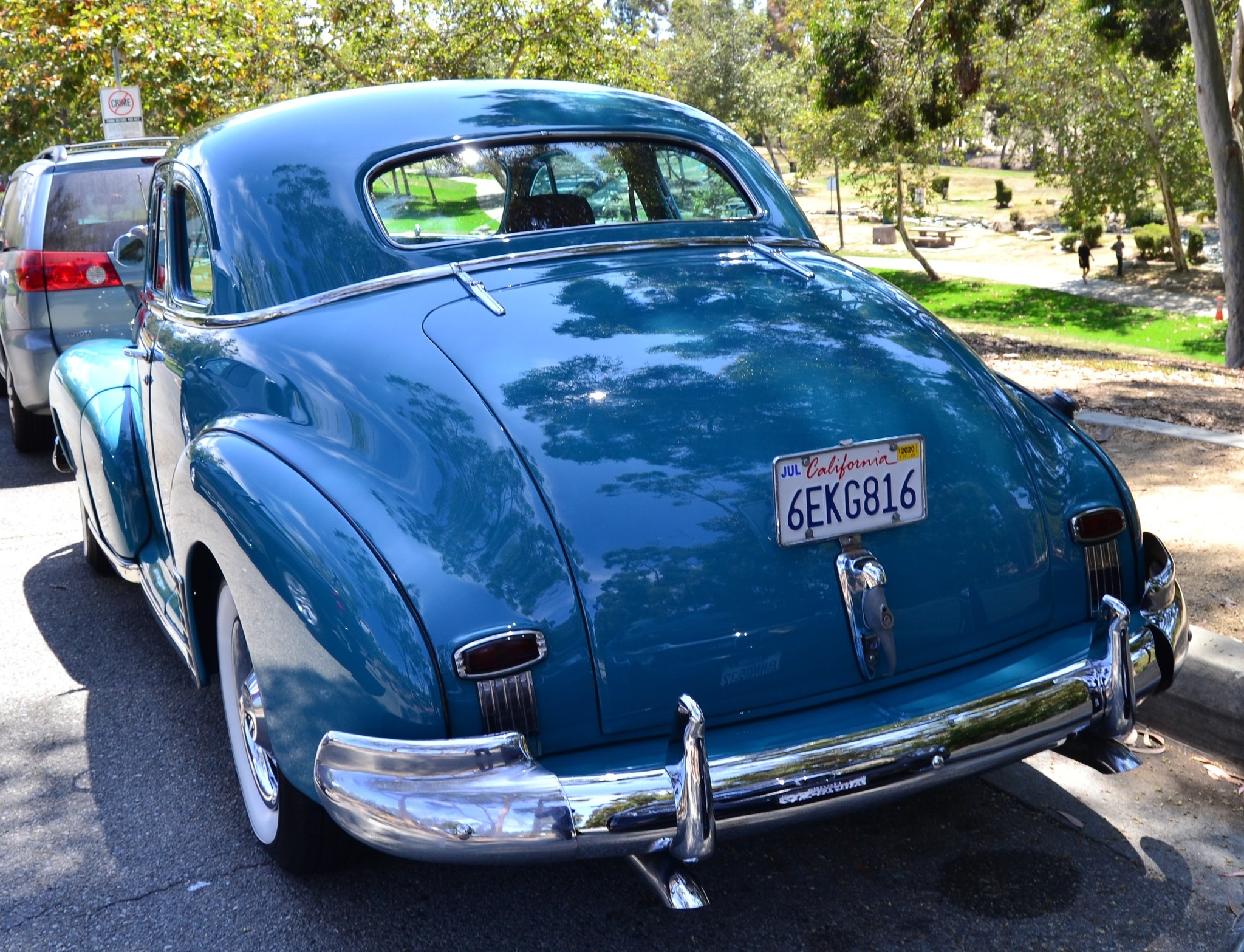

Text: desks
xmin=909 ymin=227 xmax=956 ymax=247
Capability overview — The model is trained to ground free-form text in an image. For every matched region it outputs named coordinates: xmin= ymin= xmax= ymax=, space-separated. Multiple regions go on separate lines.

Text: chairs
xmin=504 ymin=194 xmax=596 ymax=235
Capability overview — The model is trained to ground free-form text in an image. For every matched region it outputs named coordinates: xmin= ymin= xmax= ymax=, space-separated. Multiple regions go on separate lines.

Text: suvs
xmin=0 ymin=132 xmax=181 ymax=458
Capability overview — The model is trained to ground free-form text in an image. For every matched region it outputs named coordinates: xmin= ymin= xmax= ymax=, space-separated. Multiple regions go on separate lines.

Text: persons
xmin=1078 ymin=237 xmax=1094 ymax=283
xmin=1111 ymin=235 xmax=1124 ymax=278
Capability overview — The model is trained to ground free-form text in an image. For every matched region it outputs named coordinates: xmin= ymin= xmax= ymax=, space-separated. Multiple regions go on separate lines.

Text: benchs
xmin=928 ymin=235 xmax=964 ymax=245
xmin=909 ymin=235 xmax=942 ymax=247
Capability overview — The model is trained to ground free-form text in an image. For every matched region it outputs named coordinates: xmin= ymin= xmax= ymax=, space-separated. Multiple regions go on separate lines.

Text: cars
xmin=46 ymin=72 xmax=1195 ymax=915
